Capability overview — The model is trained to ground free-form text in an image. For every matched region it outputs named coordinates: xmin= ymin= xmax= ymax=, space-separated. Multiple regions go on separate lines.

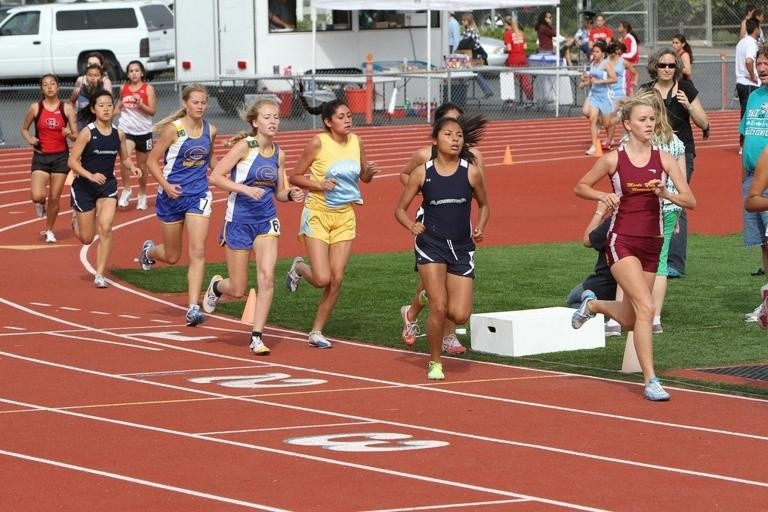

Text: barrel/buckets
xmin=265 ymin=90 xmax=293 ymax=118
xmin=344 ymin=88 xmax=367 ymax=114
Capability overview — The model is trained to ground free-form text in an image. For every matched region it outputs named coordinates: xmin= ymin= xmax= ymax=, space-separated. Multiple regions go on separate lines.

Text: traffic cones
xmin=591 ymin=138 xmax=602 ymax=157
xmin=502 ymin=144 xmax=514 ymax=166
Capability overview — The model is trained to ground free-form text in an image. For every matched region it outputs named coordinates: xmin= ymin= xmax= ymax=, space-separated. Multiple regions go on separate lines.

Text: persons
xmin=65 ymin=90 xmax=144 ymax=291
xmin=535 ymin=10 xmax=556 ymax=54
xmin=137 ymin=82 xmax=233 ymax=329
xmin=566 ymin=97 xmax=672 ymax=406
xmin=735 ymin=4 xmax=767 ymax=329
xmin=636 ymin=47 xmax=709 ymax=280
xmin=397 ymin=100 xmax=488 ymax=357
xmin=445 ymin=9 xmax=495 ymax=99
xmin=67 ymin=66 xmax=106 ymax=140
xmin=109 ymin=58 xmax=158 ymax=213
xmin=200 ymin=97 xmax=306 ymax=360
xmin=282 ymin=81 xmax=382 ymax=351
xmin=18 ymin=74 xmax=78 ymax=246
xmin=503 ymin=16 xmax=534 ymax=107
xmin=574 ymin=12 xmax=641 ymax=157
xmin=70 ymin=52 xmax=113 ymax=130
xmin=672 ymin=34 xmax=693 ymax=78
xmin=269 ymin=0 xmax=294 ymax=33
xmin=394 ymin=112 xmax=499 ymax=383
xmin=567 ymin=87 xmax=686 ymax=337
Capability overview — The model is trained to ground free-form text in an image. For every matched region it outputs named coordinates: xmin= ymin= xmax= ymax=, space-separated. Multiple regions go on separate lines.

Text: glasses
xmin=657 ymin=62 xmax=676 ymax=69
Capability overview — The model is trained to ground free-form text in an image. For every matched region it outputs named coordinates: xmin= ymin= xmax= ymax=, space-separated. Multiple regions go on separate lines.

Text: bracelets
xmin=286 ymin=189 xmax=296 ymax=203
xmin=595 ymin=211 xmax=604 ymax=216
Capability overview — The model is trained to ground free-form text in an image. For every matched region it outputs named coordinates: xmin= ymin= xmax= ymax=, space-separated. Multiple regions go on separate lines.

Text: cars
xmin=477 ymin=37 xmax=511 ymax=78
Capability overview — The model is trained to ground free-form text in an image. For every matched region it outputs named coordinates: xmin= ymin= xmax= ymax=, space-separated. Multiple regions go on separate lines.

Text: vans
xmin=0 ymin=1 xmax=176 ymax=83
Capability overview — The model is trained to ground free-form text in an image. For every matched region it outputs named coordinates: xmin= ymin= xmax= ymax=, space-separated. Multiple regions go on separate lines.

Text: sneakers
xmin=586 ymin=145 xmax=597 ymax=155
xmin=139 ymin=241 xmax=156 ymax=271
xmin=34 ymin=201 xmax=47 ymax=217
xmin=442 ymin=335 xmax=468 ymax=356
xmin=572 ymin=289 xmax=599 ymax=330
xmin=398 ymin=307 xmax=420 ymax=343
xmin=742 ymin=303 xmax=764 ymax=322
xmin=94 ymin=276 xmax=107 ymax=289
xmin=118 ymin=189 xmax=148 ymax=211
xmin=306 ymin=330 xmax=331 ymax=349
xmin=185 ymin=275 xmax=223 ymax=329
xmin=248 ymin=336 xmax=272 ymax=355
xmin=423 ymin=360 xmax=446 ymax=381
xmin=287 ymin=257 xmax=305 ymax=294
xmin=603 ymin=324 xmax=623 ymax=337
xmin=652 ymin=322 xmax=664 ymax=335
xmin=642 ymin=380 xmax=672 ymax=403
xmin=43 ymin=232 xmax=58 ymax=244
